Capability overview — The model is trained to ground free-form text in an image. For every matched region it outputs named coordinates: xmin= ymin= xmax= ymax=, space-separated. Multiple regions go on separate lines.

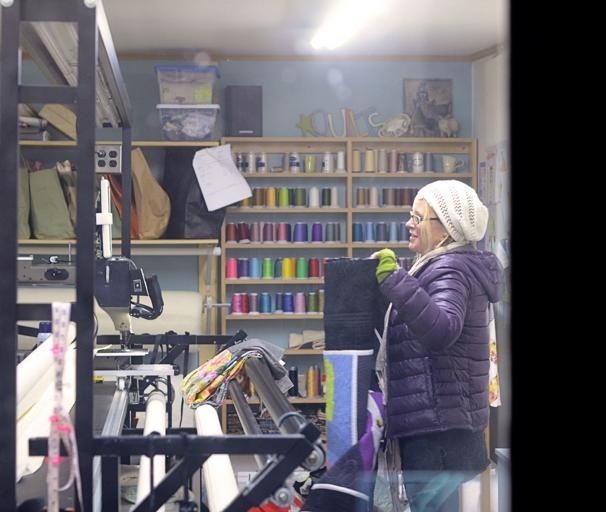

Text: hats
xmin=417 ymin=179 xmax=490 ymax=243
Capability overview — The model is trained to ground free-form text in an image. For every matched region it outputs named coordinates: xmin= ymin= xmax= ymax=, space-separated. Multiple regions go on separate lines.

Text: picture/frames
xmin=402 ymin=76 xmax=454 ymax=138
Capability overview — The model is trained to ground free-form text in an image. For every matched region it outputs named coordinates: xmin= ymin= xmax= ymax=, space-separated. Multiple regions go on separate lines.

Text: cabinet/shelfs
xmin=17 ymin=139 xmax=220 ymax=247
xmin=350 ymin=136 xmax=478 ymax=250
xmin=220 ymin=136 xmax=350 ymax=441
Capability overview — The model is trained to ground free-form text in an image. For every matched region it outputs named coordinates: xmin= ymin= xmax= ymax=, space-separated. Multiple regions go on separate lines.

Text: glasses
xmin=408 ymin=210 xmax=439 ymax=225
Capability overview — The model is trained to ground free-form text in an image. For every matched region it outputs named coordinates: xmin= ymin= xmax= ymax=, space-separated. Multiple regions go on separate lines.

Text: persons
xmin=370 ymin=179 xmax=505 ymax=512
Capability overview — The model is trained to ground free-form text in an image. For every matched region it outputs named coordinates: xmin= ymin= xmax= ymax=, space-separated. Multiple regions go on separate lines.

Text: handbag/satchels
xmin=162 ymin=147 xmax=220 ymax=240
xmin=130 ymin=147 xmax=172 ymax=240
xmin=16 ymin=153 xmax=32 ymax=241
xmin=27 ymin=157 xmax=77 ymax=241
xmin=105 ymin=174 xmax=140 ymax=240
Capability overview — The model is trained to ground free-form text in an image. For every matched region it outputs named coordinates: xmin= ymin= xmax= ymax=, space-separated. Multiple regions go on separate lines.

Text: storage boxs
xmin=157 ymin=65 xmax=222 ymax=104
xmin=155 ymin=104 xmax=221 ymax=142
